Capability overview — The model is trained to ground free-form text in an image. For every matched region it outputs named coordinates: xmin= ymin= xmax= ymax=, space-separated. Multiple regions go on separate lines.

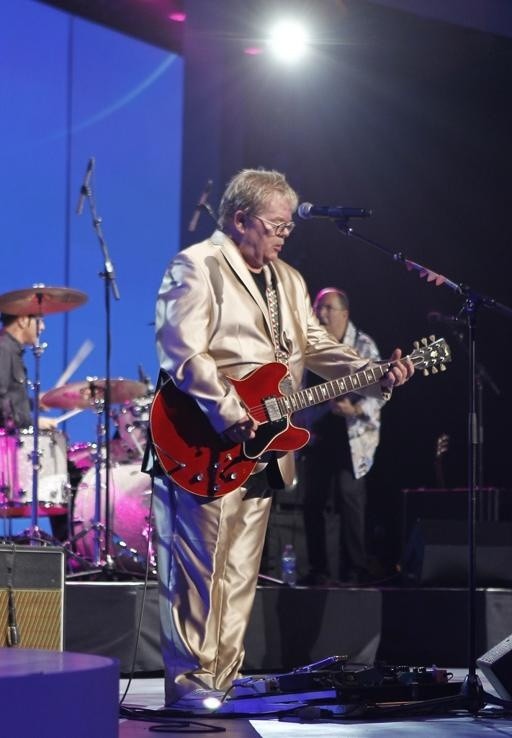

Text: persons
xmin=142 ymin=168 xmax=415 ymax=713
xmin=0 ymin=315 xmax=82 ymax=541
xmin=296 ymin=287 xmax=385 ymax=588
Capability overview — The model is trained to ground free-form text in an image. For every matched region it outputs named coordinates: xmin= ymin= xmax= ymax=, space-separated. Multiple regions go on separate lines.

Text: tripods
xmin=51 ymin=404 xmax=157 ymax=583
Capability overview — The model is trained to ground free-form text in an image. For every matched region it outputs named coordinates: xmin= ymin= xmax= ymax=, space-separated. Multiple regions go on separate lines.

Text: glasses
xmin=242 ymin=210 xmax=296 ymax=236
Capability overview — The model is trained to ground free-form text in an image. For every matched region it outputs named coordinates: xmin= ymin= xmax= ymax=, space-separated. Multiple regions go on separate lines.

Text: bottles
xmin=281 ymin=545 xmax=297 ymax=586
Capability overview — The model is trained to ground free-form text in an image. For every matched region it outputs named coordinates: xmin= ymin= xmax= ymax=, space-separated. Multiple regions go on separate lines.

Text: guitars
xmin=148 ymin=334 xmax=451 ymax=502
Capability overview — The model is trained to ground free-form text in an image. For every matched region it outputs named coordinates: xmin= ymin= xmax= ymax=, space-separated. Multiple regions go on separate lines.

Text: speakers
xmin=1 ymin=551 xmax=68 ymax=653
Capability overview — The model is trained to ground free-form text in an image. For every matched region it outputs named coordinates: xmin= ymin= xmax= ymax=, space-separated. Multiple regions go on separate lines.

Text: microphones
xmin=186 ymin=175 xmax=215 ymax=234
xmin=295 ymin=200 xmax=375 ymax=227
xmin=428 ymin=309 xmax=464 ymax=335
xmin=74 ymin=154 xmax=98 ymax=217
xmin=4 ymin=579 xmax=20 ymax=649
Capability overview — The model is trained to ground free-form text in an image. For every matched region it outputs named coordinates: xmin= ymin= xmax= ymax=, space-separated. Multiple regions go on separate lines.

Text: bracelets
xmin=353 ymin=408 xmax=358 ymax=421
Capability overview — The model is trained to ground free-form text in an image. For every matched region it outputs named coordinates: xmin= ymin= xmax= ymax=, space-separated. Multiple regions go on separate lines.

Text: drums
xmin=68 ymin=458 xmax=155 ymax=579
xmin=115 ymin=393 xmax=156 ymax=452
xmin=67 ymin=439 xmax=131 ymax=473
xmin=0 ymin=426 xmax=70 ymax=518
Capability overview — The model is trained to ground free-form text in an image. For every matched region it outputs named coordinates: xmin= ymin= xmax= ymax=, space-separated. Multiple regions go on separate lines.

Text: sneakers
xmin=233 ymin=677 xmax=267 ymax=696
xmin=298 ymin=569 xmax=373 ymax=586
xmin=167 ymin=686 xmax=235 ymax=714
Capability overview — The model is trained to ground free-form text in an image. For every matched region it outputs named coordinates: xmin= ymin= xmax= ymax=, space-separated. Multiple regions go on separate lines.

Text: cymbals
xmin=0 ymin=286 xmax=88 ymax=316
xmin=40 ymin=375 xmax=152 ymax=409
